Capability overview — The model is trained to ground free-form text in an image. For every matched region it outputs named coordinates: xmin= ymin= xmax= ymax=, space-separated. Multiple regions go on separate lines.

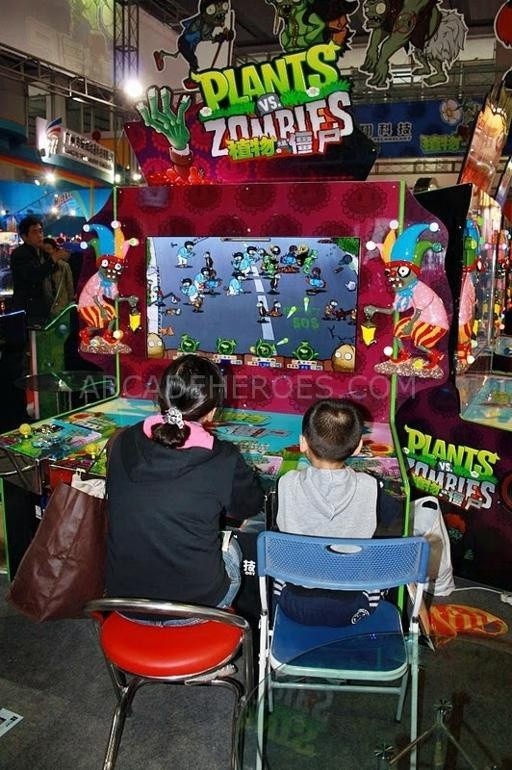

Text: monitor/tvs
xmin=142 ymin=227 xmax=366 ymax=375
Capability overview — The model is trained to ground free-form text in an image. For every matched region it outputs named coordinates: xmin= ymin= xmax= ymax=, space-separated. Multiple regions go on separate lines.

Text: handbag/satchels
xmin=4 ymin=424 xmax=130 ymax=623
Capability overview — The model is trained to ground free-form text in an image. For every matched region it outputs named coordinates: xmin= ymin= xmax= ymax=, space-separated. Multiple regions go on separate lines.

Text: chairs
xmin=86 ymin=595 xmax=253 ymax=768
xmin=253 ymin=531 xmax=429 ymax=769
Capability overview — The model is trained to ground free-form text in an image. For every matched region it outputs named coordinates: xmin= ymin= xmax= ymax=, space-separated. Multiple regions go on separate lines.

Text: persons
xmin=8 ymin=215 xmax=60 ymax=328
xmin=270 ymin=397 xmax=409 ymax=630
xmin=101 ymin=354 xmax=264 ymax=630
xmin=35 ymin=238 xmax=81 ymax=323
